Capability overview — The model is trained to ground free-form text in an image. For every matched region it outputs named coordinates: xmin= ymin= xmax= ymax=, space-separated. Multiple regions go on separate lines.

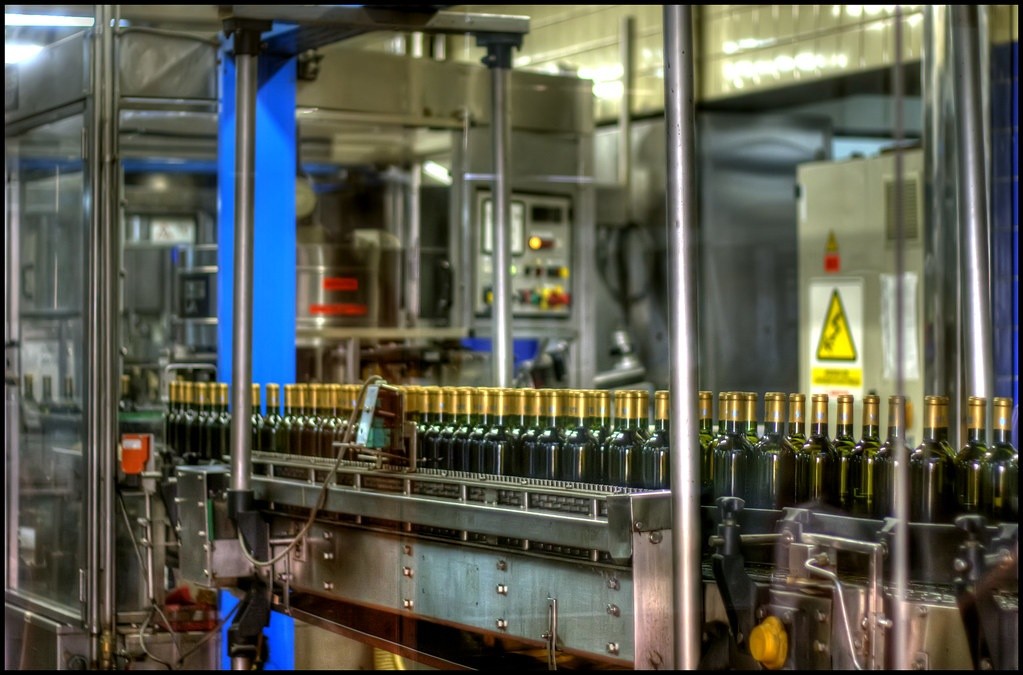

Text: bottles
xmin=55 ymin=378 xmax=79 ymax=419
xmin=119 ymin=376 xmax=136 ymax=412
xmin=21 ymin=374 xmax=37 ymax=412
xmin=38 ymin=376 xmax=57 ymax=416
xmin=162 ymin=379 xmax=1019 ymax=531
